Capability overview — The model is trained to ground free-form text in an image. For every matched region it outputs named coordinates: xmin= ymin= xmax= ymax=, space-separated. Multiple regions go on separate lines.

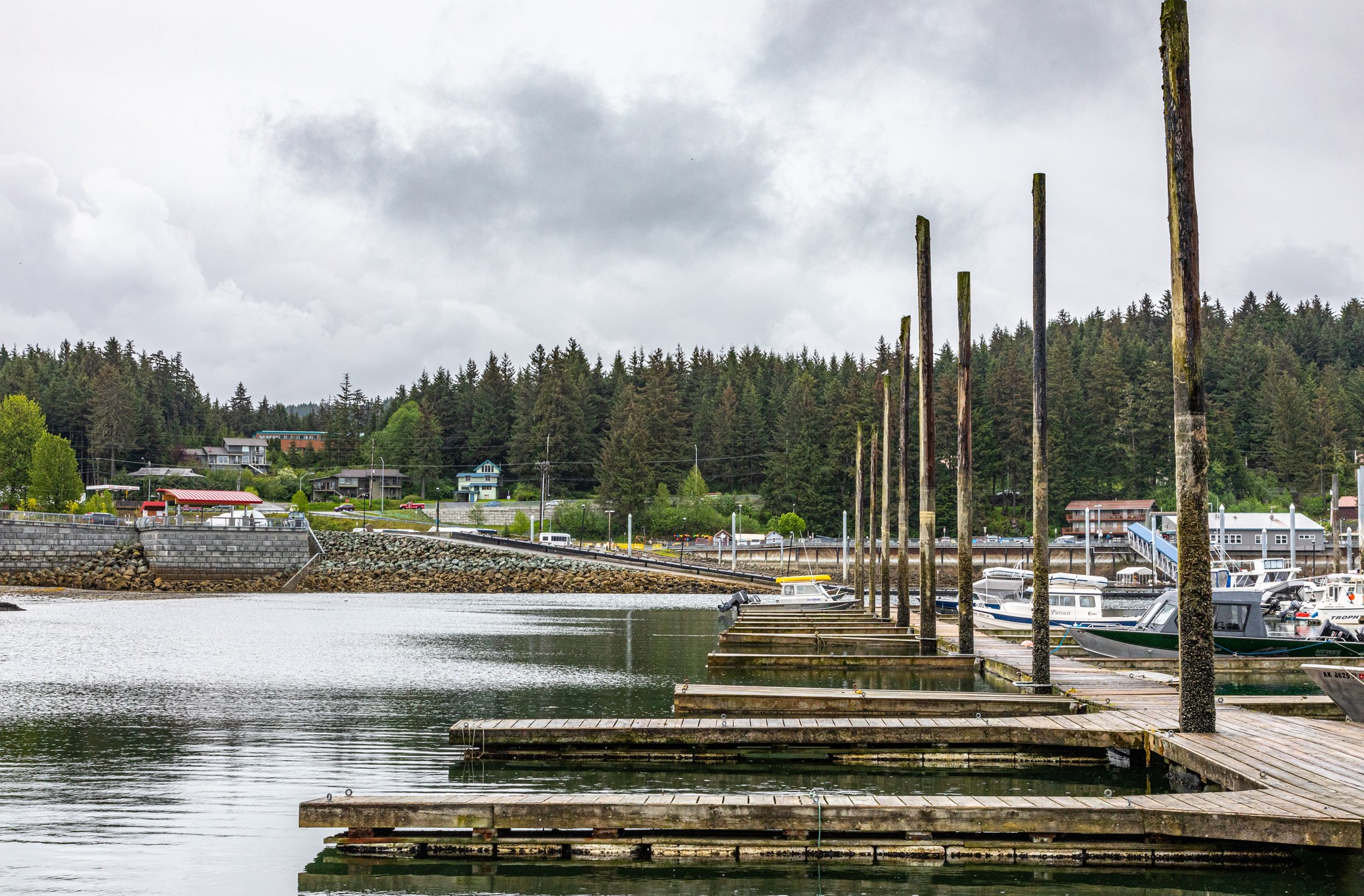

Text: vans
xmin=482 ymin=501 xmax=502 ymax=507
xmin=540 ymin=532 xmax=573 ymax=548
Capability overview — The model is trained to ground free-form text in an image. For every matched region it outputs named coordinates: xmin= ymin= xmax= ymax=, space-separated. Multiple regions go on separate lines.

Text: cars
xmin=854 ymin=533 xmax=1077 ymax=544
xmin=177 ymin=504 xmax=235 ymax=512
xmin=810 ymin=538 xmax=822 ymax=543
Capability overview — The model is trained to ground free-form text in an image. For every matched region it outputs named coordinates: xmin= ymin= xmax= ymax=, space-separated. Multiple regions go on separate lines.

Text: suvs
xmin=82 ymin=512 xmax=126 ymax=526
xmin=334 ymin=504 xmax=356 ymax=512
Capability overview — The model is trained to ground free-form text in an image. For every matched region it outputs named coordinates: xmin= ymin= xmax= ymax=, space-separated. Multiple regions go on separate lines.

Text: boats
xmin=916 ymin=558 xmax=1364 ymax=722
xmin=716 ymin=530 xmax=862 ymax=614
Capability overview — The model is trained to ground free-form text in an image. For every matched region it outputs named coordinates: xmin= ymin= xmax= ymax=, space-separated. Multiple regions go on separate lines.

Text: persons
xmin=1110 ymin=535 xmax=1115 ymax=543
xmin=1027 ymin=535 xmax=1030 ymax=543
xmin=1115 ymin=515 xmax=1117 ymax=519
xmin=1134 ymin=575 xmax=1137 ymax=584
xmin=1106 ymin=534 xmax=1110 ymax=543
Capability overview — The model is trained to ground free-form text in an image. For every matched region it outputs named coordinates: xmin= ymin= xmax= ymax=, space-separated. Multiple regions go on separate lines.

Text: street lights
xmin=436 ymin=487 xmax=440 ymax=532
xmin=680 ymin=518 xmax=687 ymax=564
xmin=359 ymin=487 xmax=366 ymax=532
xmin=605 ymin=510 xmax=615 ymax=550
xmin=1094 ymin=504 xmax=1102 ymax=544
xmin=22 ymin=486 xmax=30 ymax=512
xmin=786 ymin=531 xmax=794 ymax=578
xmin=379 ymin=457 xmax=384 ymax=512
xmin=151 ymin=495 xmax=160 ymax=502
xmin=739 ymin=504 xmax=743 ymax=546
xmin=299 ymin=473 xmax=315 ymax=492
xmin=141 ymin=458 xmax=151 ymax=502
xmin=578 ymin=504 xmax=586 ymax=551
xmin=1340 ymin=517 xmax=1346 ymax=545
xmin=793 ymin=504 xmax=795 ymax=513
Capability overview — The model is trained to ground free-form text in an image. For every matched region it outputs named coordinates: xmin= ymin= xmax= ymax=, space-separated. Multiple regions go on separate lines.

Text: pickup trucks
xmin=400 ymin=501 xmax=425 ymax=510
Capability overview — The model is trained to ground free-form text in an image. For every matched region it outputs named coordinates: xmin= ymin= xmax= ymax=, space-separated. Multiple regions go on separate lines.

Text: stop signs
xmin=345 ymin=499 xmax=349 ymax=503
